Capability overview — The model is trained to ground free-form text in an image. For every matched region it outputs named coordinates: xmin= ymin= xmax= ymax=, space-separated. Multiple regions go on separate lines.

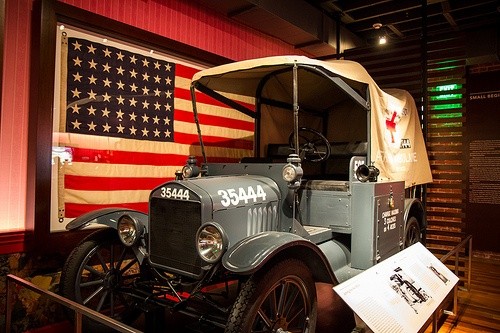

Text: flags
xmin=58 ymin=30 xmax=256 ymax=229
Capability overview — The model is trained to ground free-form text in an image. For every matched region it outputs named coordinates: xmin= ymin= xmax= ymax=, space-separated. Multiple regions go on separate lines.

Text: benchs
xmin=273 ymin=152 xmax=367 ymax=192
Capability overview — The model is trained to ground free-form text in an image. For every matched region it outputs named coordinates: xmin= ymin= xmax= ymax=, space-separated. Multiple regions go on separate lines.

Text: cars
xmin=61 ymin=54 xmax=436 ymax=332
